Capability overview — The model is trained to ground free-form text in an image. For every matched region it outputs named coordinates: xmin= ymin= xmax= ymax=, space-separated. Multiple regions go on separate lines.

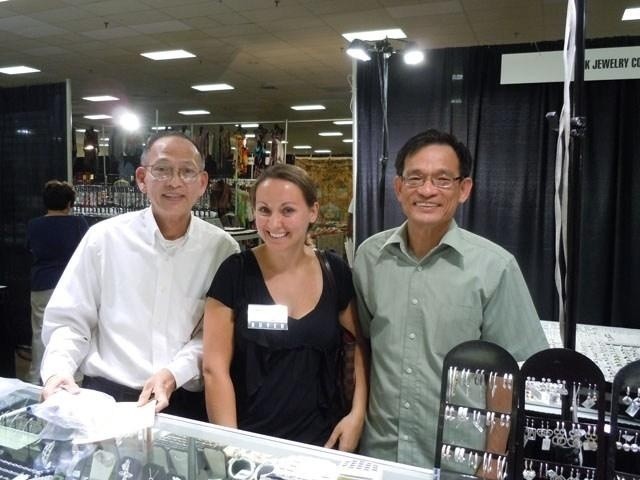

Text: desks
xmin=0 ymin=377 xmax=433 ymax=480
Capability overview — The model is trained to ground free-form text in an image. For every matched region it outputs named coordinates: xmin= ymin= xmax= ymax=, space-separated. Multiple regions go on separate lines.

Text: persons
xmin=239 ymin=181 xmax=253 ymax=230
xmin=38 ymin=131 xmax=240 ymax=422
xmin=353 ymin=128 xmax=549 ymax=479
xmin=99 ymin=190 xmax=112 ymax=208
xmin=24 ymin=180 xmax=91 ymax=387
xmin=202 ymin=162 xmax=368 ymax=453
xmin=83 ymin=125 xmax=286 ymax=178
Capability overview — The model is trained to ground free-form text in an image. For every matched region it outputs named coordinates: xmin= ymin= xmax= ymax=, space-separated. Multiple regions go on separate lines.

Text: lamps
xmin=345 ymin=35 xmax=424 ymax=66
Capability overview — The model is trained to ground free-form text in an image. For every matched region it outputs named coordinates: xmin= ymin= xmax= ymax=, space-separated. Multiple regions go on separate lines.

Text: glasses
xmin=145 ymin=164 xmax=202 ymax=184
xmin=400 ymin=175 xmax=461 ymax=188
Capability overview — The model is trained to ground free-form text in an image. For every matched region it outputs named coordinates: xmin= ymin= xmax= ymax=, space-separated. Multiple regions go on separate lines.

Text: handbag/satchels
xmin=334 ymin=324 xmax=356 ymax=400
